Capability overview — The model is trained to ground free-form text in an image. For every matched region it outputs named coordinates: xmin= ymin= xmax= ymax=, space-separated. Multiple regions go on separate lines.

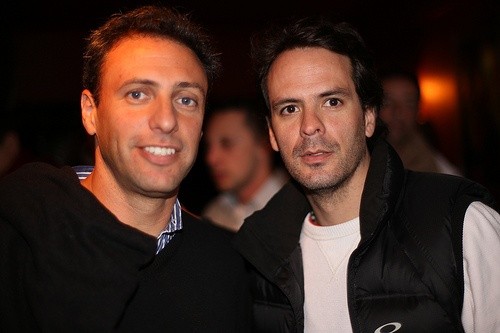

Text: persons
xmin=229 ymin=16 xmax=500 ymax=333
xmin=0 ymin=91 xmax=64 ymax=201
xmin=371 ymin=62 xmax=463 ymax=179
xmin=1 ymin=3 xmax=256 ymax=332
xmin=198 ymin=89 xmax=293 ymax=233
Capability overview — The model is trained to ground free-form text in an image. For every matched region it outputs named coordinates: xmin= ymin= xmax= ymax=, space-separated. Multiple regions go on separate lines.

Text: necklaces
xmin=308 ymin=209 xmax=321 ymax=225
